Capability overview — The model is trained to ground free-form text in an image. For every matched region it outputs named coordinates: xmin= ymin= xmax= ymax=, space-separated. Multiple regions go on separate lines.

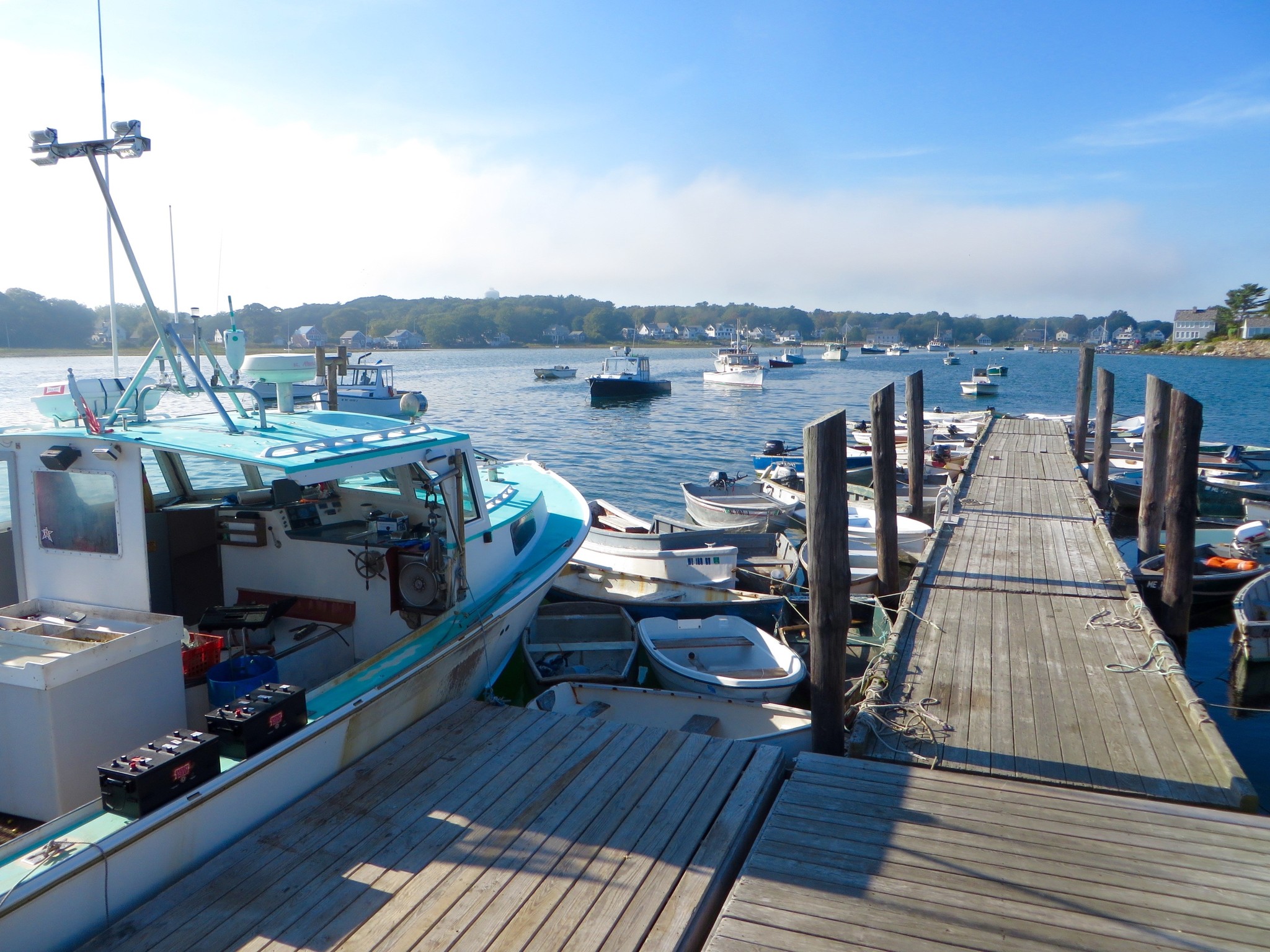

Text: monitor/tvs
xmin=272 ymin=478 xmax=302 ymax=506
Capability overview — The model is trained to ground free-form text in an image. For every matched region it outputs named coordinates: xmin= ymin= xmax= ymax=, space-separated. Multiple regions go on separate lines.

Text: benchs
xmin=576 ymin=700 xmax=610 ymax=718
xmin=777 ymin=634 xmax=885 ymax=646
xmin=629 ymin=588 xmax=684 ymax=601
xmin=1194 ymin=556 xmax=1248 ymax=572
xmin=651 ymin=636 xmax=753 ymax=649
xmin=680 ymin=714 xmax=719 ymax=734
xmin=897 ymin=484 xmax=947 ymax=502
xmin=849 ymin=549 xmax=878 ymax=575
xmin=700 ymin=495 xmax=781 ymax=507
xmin=848 ymin=514 xmax=868 ymax=525
xmin=598 ymin=515 xmax=643 ymax=533
xmin=526 ymin=641 xmax=635 ymax=652
xmin=538 ymin=613 xmax=624 ymax=619
xmin=736 ymin=559 xmax=793 ymax=566
xmin=1252 ymin=599 xmax=1270 ymax=607
xmin=706 ymin=668 xmax=788 ymax=679
xmin=1238 ymin=483 xmax=1270 ymax=489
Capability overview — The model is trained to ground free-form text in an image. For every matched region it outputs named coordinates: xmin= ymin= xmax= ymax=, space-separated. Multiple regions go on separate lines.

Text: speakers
xmin=40 ymin=446 xmax=77 ymax=470
xmin=91 ymin=445 xmax=121 ymax=461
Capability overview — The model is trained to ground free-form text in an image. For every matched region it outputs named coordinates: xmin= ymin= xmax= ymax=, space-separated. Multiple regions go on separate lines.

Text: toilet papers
xmin=237 ymin=488 xmax=274 ymax=506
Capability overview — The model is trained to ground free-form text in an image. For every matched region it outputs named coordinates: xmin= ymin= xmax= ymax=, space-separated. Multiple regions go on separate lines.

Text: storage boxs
xmin=29 ymin=377 xmax=159 ymax=418
xmin=181 ymin=633 xmax=225 ymax=675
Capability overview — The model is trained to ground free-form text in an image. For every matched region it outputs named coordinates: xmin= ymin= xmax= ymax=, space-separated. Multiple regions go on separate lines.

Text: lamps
xmin=92 ymin=445 xmax=120 ymax=461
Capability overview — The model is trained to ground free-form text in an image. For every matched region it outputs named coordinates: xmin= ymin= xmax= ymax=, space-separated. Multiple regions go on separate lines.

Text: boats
xmin=861 ymin=340 xmax=886 ymax=354
xmin=520 ymin=601 xmax=642 ymax=689
xmin=1004 ymin=343 xmax=1015 ymax=350
xmin=768 ymin=357 xmax=794 ymax=369
xmin=533 ymin=365 xmax=579 ymax=379
xmin=781 ymin=345 xmax=807 ymax=365
xmin=542 ymin=405 xmax=993 ymax=661
xmin=247 ymin=379 xmax=327 ymax=401
xmin=634 ymin=612 xmax=807 ymax=705
xmin=585 ymin=314 xmax=673 ymax=402
xmin=311 ymin=352 xmax=428 ymax=418
xmin=1023 ymin=344 xmax=1034 ymax=351
xmin=240 ymin=311 xmax=316 ymax=383
xmin=1023 ymin=411 xmax=1269 ymax=666
xmin=821 ymin=334 xmax=849 ymax=361
xmin=1 ymin=116 xmax=592 ymax=952
xmin=986 ymin=357 xmax=1009 ymax=376
xmin=886 ymin=341 xmax=909 ymax=356
xmin=960 ymin=367 xmax=1000 ymax=397
xmin=703 ymin=317 xmax=770 ymax=387
xmin=969 ymin=350 xmax=978 ymax=355
xmin=525 ymin=680 xmax=813 ymax=779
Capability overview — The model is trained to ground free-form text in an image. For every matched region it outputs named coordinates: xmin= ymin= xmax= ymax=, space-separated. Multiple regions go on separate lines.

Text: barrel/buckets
xmin=206 ymin=654 xmax=280 ymax=712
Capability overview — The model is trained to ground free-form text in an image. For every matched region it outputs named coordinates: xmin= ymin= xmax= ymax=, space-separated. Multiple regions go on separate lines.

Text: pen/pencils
xmin=288 ymin=624 xmax=310 ymax=633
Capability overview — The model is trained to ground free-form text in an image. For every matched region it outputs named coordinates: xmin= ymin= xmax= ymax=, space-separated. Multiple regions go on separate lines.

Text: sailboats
xmin=926 ymin=321 xmax=951 ymax=352
xmin=942 ymin=339 xmax=963 ymax=366
xmin=30 ymin=0 xmax=173 ymax=425
xmin=1094 ymin=318 xmax=1140 ymax=355
xmin=1038 ymin=320 xmax=1061 ymax=354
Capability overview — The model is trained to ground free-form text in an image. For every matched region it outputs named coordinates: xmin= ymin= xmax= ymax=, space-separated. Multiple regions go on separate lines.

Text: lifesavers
xmin=979 ymin=381 xmax=986 ymax=383
